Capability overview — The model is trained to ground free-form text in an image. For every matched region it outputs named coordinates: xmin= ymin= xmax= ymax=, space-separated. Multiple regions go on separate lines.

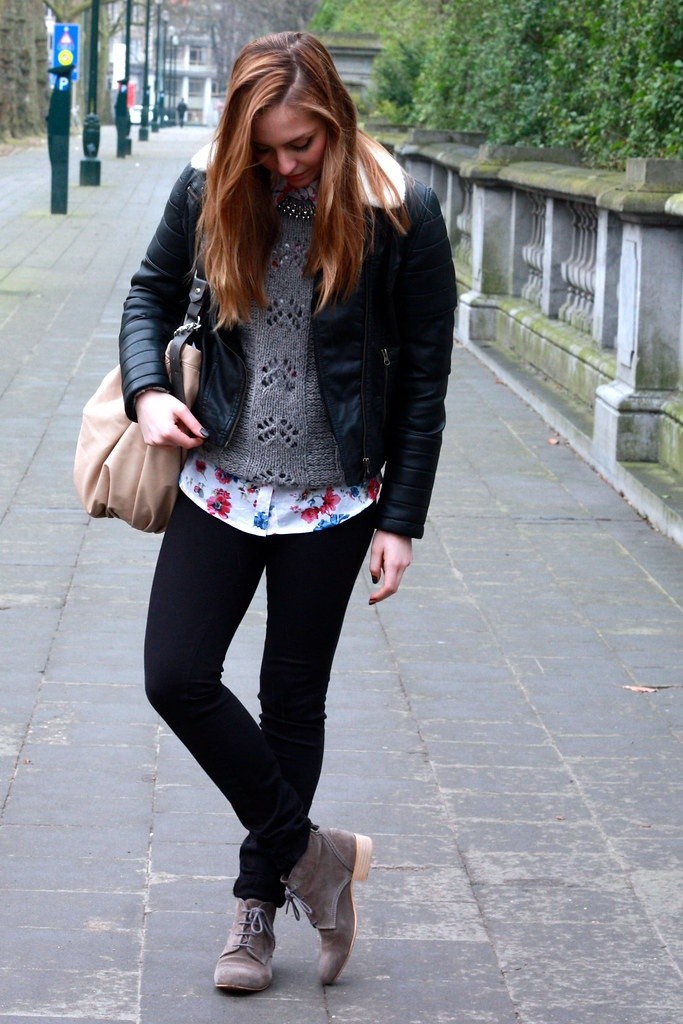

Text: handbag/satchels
xmin=73 ymin=226 xmax=208 ymax=535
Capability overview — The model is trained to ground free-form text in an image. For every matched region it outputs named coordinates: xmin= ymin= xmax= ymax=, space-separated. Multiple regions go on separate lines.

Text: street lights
xmin=159 ymin=8 xmax=170 ymax=128
xmin=151 ymin=0 xmax=164 ymax=133
xmin=164 ymin=25 xmax=179 ymax=126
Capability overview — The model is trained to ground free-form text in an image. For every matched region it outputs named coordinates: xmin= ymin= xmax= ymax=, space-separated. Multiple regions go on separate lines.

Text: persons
xmin=118 ymin=31 xmax=462 ymax=996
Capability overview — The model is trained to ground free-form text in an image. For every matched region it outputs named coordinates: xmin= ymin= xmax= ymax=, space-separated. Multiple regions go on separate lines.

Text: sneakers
xmin=285 ymin=826 xmax=374 ymax=984
xmin=213 ymin=895 xmax=277 ymax=990
